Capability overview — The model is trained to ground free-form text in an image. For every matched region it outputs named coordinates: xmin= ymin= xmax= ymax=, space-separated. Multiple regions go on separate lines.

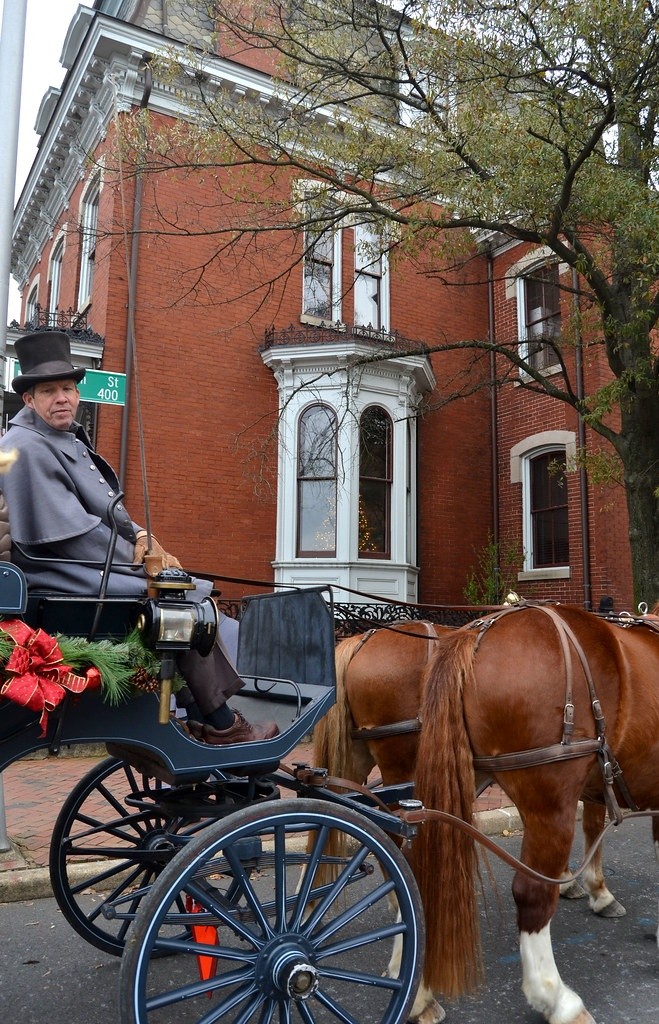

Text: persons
xmin=0 ymin=332 xmax=282 ymax=747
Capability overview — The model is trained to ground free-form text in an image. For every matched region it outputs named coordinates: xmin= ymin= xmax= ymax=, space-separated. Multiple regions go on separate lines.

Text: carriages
xmin=0 ymin=492 xmax=659 ymax=1024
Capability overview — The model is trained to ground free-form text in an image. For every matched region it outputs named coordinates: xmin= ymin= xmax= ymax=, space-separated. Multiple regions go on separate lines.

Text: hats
xmin=12 ymin=332 xmax=86 ymax=396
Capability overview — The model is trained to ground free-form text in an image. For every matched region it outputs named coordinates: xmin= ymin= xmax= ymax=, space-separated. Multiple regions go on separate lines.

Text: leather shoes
xmin=186 ymin=707 xmax=281 ymax=746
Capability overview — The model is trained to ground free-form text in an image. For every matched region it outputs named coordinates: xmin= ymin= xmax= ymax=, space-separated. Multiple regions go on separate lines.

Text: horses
xmin=294 ymin=621 xmax=627 ymax=933
xmin=387 ymin=602 xmax=658 ymax=1024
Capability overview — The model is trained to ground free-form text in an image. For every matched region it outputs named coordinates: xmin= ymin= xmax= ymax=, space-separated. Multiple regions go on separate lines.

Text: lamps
xmin=139 ymin=595 xmax=219 ymax=658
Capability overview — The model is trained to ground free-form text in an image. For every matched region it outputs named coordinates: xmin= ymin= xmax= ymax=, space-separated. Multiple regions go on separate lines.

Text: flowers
xmin=0 ymin=618 xmax=101 ymax=740
